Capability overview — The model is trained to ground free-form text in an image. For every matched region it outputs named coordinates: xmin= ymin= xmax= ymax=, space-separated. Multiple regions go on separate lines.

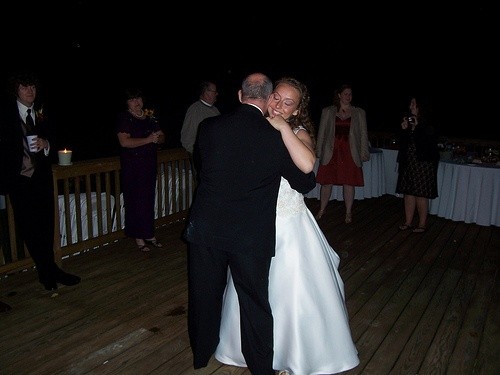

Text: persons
xmin=180 ymin=81 xmax=221 ymax=174
xmin=116 ymin=87 xmax=166 ymax=251
xmin=181 ymin=73 xmax=317 ymax=375
xmin=315 ymin=85 xmax=370 ymax=225
xmin=395 ymin=97 xmax=439 ymax=233
xmin=214 ymin=77 xmax=361 ymax=375
xmin=0 ymin=74 xmax=81 ymax=290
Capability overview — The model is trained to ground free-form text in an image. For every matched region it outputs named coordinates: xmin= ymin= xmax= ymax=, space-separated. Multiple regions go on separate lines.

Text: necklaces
xmin=129 ymin=108 xmax=144 ymax=117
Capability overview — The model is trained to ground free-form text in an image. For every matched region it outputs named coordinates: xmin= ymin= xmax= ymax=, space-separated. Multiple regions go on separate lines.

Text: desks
xmin=303 ymin=147 xmax=500 ymax=227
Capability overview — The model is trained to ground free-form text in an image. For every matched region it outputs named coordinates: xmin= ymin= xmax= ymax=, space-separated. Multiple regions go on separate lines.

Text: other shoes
xmin=0 ymin=302 xmax=12 ymax=312
xmin=399 ymin=223 xmax=415 ymax=231
xmin=412 ymin=225 xmax=427 ymax=233
xmin=314 ymin=212 xmax=326 ymax=221
xmin=136 ymin=243 xmax=151 ymax=252
xmin=38 ymin=263 xmax=81 ymax=290
xmin=343 ymin=214 xmax=353 ymax=225
xmin=145 ymin=238 xmax=163 ymax=248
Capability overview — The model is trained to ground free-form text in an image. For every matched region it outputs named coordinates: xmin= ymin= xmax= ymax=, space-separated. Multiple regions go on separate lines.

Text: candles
xmin=58 ymin=148 xmax=72 ymax=165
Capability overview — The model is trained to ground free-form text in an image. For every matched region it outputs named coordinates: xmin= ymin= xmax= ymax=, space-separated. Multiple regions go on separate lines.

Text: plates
xmin=57 ymin=163 xmax=72 ymax=166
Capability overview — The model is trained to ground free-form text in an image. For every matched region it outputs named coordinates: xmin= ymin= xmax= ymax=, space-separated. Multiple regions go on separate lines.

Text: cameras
xmin=406 ymin=117 xmax=415 ymax=123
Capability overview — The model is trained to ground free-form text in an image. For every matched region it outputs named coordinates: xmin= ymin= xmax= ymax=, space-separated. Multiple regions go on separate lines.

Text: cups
xmin=26 ymin=135 xmax=38 ymax=152
xmin=488 ymin=144 xmax=496 ymax=158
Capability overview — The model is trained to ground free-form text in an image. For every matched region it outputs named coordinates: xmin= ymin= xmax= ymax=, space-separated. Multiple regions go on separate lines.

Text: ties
xmin=26 ymin=109 xmax=38 ymax=164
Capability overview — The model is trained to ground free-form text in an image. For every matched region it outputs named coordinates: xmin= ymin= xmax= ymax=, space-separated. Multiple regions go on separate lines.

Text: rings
xmin=271 ymin=115 xmax=276 ymax=119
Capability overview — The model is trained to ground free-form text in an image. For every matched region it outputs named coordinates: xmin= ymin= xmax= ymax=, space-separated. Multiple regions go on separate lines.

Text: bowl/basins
xmin=481 ymin=156 xmax=499 ymax=163
